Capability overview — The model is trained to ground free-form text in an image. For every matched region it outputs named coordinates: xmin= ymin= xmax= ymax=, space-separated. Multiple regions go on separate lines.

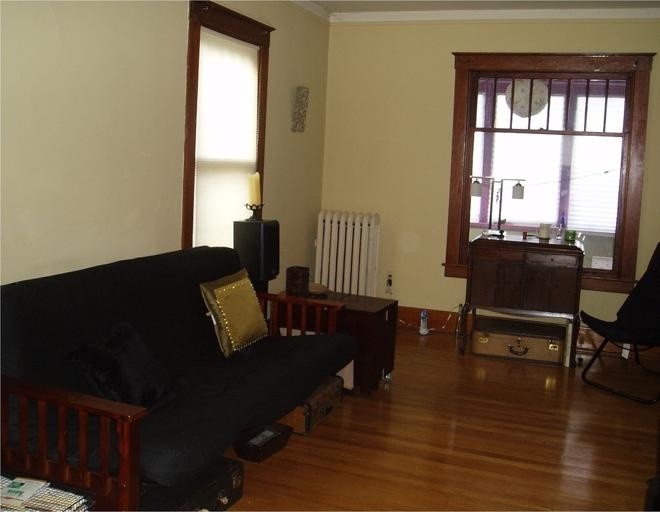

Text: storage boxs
xmin=276 ymin=374 xmax=344 ymax=437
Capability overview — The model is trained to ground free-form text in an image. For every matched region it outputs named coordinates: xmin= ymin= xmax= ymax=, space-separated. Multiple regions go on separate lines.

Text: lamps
xmin=292 ymin=85 xmax=310 ymax=134
xmin=469 ymin=175 xmax=527 ymax=233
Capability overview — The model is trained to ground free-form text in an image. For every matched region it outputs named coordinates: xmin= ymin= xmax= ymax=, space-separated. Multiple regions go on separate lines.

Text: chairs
xmin=579 ymin=239 xmax=660 ymax=405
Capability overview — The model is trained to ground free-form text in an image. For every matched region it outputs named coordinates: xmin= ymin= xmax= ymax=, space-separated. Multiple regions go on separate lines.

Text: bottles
xmin=418 ymin=310 xmax=429 ymax=336
xmin=523 ymin=232 xmax=528 ymax=240
xmin=555 ymin=225 xmax=562 ymax=240
xmin=538 ymin=223 xmax=552 ymax=239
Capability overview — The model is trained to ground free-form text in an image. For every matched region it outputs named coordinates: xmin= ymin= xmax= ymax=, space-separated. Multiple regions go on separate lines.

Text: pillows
xmin=194 ymin=268 xmax=272 ymax=360
xmin=66 ymin=328 xmax=176 ymax=421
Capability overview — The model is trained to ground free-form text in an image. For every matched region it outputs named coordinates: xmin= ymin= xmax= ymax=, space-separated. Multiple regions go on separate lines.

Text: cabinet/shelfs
xmin=275 ymin=288 xmax=398 ymax=390
xmin=459 ymin=232 xmax=584 ymax=370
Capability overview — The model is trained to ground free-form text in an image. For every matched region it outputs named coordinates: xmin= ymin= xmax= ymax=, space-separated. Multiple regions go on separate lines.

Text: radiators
xmin=314 ymin=208 xmax=381 ymax=299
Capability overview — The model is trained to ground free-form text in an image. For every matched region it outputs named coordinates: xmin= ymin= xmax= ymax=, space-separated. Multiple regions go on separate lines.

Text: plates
xmin=309 ymin=283 xmax=329 ymax=295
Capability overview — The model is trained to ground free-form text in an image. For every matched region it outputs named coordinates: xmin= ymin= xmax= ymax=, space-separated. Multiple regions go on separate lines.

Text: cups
xmin=286 ymin=265 xmax=310 ymax=296
xmin=564 ymin=229 xmax=576 ymax=242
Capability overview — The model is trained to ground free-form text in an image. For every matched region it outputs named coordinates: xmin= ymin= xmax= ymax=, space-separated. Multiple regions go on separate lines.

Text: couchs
xmin=2 ymin=245 xmax=358 ymax=511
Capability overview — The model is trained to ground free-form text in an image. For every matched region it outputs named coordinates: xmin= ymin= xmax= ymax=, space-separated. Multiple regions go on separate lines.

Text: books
xmin=2 ymin=477 xmax=48 ymax=506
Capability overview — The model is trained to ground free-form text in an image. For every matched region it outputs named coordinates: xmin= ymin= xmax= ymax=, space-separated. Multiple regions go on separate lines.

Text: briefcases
xmin=467 ymin=315 xmax=570 ymax=365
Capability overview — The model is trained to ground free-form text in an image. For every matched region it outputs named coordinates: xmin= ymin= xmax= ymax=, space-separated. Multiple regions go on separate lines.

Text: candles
xmin=246 ymin=171 xmax=262 ymax=207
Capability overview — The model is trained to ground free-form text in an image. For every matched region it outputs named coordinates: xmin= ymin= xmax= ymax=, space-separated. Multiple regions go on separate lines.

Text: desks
xmin=3 ymin=466 xmax=96 ymax=511
